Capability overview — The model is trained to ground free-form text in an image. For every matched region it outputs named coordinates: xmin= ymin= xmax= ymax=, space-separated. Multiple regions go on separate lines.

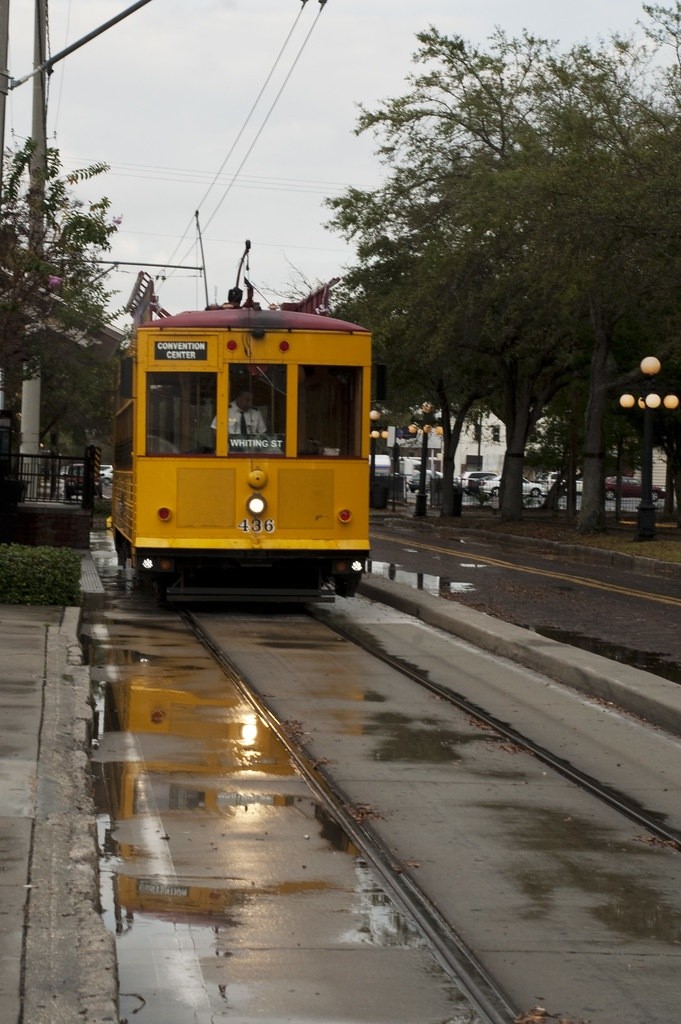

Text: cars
xmin=408 ymin=471 xmax=440 ymax=493
xmin=99 ymin=465 xmax=113 ymax=485
xmin=64 ymin=464 xmax=106 ymax=500
xmin=605 ymin=476 xmax=666 ymax=501
xmin=480 ymin=475 xmax=545 ymax=498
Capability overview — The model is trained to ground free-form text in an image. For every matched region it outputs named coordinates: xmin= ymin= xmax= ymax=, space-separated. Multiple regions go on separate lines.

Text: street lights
xmin=408 ymin=401 xmax=444 ymax=517
xmin=370 ymin=410 xmax=389 ymax=476
xmin=619 ymin=356 xmax=679 ymax=543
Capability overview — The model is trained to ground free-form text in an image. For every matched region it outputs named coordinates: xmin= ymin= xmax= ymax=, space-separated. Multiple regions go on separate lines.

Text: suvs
xmin=456 ymin=471 xmax=500 ymax=495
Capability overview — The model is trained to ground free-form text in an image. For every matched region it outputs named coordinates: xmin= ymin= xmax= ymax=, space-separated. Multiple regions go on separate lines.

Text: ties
xmin=236 ymin=410 xmax=247 ymax=438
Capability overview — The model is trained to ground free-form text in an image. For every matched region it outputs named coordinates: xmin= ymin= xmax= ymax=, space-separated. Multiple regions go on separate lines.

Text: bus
xmin=111 ymin=286 xmax=372 ymax=605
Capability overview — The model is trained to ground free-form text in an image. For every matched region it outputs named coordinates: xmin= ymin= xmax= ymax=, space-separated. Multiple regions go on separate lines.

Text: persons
xmin=210 ymin=388 xmax=267 ymax=442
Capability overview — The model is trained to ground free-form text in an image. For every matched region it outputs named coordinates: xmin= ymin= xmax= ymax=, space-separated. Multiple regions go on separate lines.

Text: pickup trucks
xmin=538 ymin=472 xmax=583 ymax=492
